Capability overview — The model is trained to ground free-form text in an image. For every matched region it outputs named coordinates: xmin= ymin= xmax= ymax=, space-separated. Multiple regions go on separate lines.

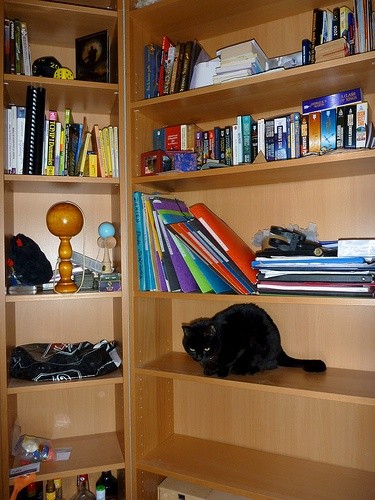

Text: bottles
xmin=25 ymin=482 xmax=38 ymax=500
xmin=96 ymin=470 xmax=118 ymax=500
xmin=45 ymin=478 xmax=62 ymax=500
xmin=72 ymin=474 xmax=96 ymax=500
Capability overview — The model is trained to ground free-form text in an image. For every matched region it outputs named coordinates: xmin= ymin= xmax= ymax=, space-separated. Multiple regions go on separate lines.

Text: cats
xmin=182 ymin=303 xmax=327 ymax=378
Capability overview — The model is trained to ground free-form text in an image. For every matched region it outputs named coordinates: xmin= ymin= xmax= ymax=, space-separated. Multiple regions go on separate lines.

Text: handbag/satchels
xmin=9 ymin=233 xmax=53 ymax=285
xmin=10 ymin=339 xmax=121 ymax=382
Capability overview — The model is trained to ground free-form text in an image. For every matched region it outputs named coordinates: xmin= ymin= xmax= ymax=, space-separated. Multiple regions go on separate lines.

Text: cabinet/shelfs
xmin=0 ymin=0 xmax=375 ymax=500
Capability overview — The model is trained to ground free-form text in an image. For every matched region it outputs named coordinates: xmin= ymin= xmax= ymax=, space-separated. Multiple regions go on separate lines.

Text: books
xmin=3 ymin=84 xmax=120 ymax=179
xmin=139 ymin=87 xmax=375 ymax=177
xmin=74 ymin=28 xmax=111 ymax=84
xmin=2 ymin=17 xmax=33 ymax=77
xmin=143 ymin=0 xmax=375 ymax=100
